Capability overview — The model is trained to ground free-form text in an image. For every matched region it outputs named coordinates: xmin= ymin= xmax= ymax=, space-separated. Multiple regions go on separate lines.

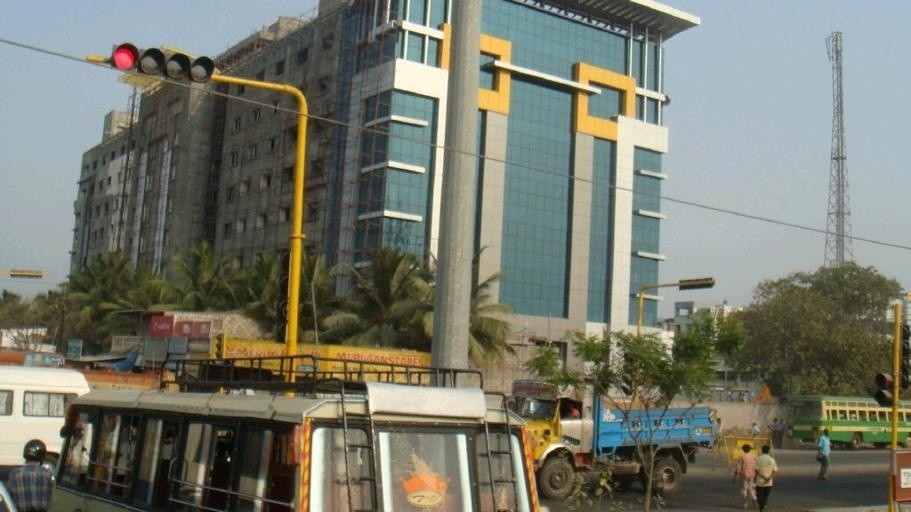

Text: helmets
xmin=24 ymin=440 xmax=46 ymax=461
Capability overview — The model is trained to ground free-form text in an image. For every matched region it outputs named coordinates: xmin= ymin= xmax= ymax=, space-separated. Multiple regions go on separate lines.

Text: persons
xmin=755 ymin=445 xmax=778 ymax=512
xmin=733 ymin=444 xmax=758 ymax=509
xmin=817 ymin=428 xmax=832 ymax=480
xmin=566 ymin=401 xmax=581 ymax=419
xmin=715 ymin=418 xmax=723 ymax=435
xmin=768 ymin=418 xmax=785 ymax=448
xmin=6 ymin=439 xmax=54 ymax=512
xmin=751 ymin=422 xmax=761 ymax=437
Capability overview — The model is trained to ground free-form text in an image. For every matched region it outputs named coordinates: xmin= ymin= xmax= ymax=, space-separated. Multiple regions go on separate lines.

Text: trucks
xmin=504 ymin=377 xmax=717 ymax=501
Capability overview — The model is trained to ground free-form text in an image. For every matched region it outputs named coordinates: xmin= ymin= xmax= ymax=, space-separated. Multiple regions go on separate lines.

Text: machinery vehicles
xmin=727 ymin=383 xmax=775 ymax=404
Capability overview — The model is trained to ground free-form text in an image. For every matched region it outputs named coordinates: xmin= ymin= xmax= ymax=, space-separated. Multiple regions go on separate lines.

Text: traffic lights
xmin=678 ymin=277 xmax=715 ymax=290
xmin=874 ymin=372 xmax=893 ymax=405
xmin=109 ymin=43 xmax=216 ymax=82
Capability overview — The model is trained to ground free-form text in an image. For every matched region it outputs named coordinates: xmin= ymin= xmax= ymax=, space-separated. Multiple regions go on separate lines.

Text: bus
xmin=788 ymin=392 xmax=910 ymax=448
xmin=0 ymin=366 xmax=91 ymax=484
xmin=48 ymin=353 xmax=545 ymax=512
